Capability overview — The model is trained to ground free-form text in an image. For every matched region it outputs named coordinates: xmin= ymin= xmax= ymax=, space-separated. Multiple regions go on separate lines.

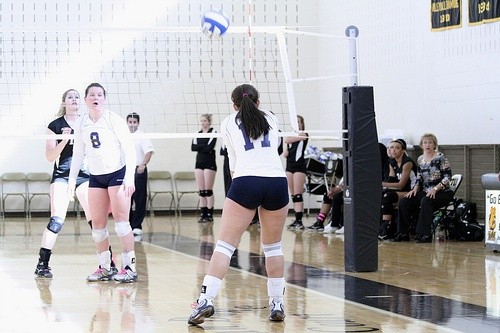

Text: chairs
xmin=175 ymin=172 xmax=201 ymax=217
xmin=147 ymin=171 xmax=176 ymax=217
xmin=430 ymin=174 xmax=464 ymax=241
xmin=0 ymin=173 xmax=28 ymax=235
xmin=303 ymin=155 xmax=343 ymax=214
xmin=27 ymin=173 xmax=52 ymax=233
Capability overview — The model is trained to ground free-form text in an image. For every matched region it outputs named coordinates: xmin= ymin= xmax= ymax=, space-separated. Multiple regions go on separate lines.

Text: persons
xmin=67 ymin=83 xmax=138 ymax=282
xmin=307 ymin=159 xmax=344 ymax=230
xmin=378 ymin=139 xmax=418 ymax=241
xmin=188 ymin=84 xmax=289 ymax=324
xmin=390 ymin=134 xmax=454 ymax=243
xmin=34 ymin=89 xmax=118 ymax=279
xmin=126 ymin=112 xmax=153 ymax=235
xmin=191 ymin=114 xmax=217 ymax=223
xmin=220 ymin=110 xmax=308 ymax=231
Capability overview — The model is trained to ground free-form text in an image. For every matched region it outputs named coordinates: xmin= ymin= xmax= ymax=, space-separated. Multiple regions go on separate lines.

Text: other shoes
xmin=377 ymin=233 xmax=395 ymax=242
xmin=323 ymin=223 xmax=340 ymax=232
xmin=287 ymin=220 xmax=304 ymax=230
xmin=336 ymin=226 xmax=344 ymax=234
xmin=198 ymin=213 xmax=213 ymax=223
xmin=306 ymin=222 xmax=324 ymax=231
xmin=133 ymin=228 xmax=143 ymax=235
xmin=415 ymin=233 xmax=433 ymax=244
xmin=390 ymin=233 xmax=411 ymax=242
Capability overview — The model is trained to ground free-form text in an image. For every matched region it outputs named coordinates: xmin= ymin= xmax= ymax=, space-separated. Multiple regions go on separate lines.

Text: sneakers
xmin=111 ymin=267 xmax=118 ymax=275
xmin=269 ymin=298 xmax=286 ymax=321
xmin=113 ymin=265 xmax=137 ymax=282
xmin=188 ymin=298 xmax=215 ymax=324
xmin=86 ymin=264 xmax=113 ymax=281
xmin=34 ymin=261 xmax=53 ymax=278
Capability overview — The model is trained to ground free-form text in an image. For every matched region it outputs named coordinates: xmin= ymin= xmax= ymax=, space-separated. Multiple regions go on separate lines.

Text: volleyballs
xmin=200 ymin=9 xmax=230 ymax=39
